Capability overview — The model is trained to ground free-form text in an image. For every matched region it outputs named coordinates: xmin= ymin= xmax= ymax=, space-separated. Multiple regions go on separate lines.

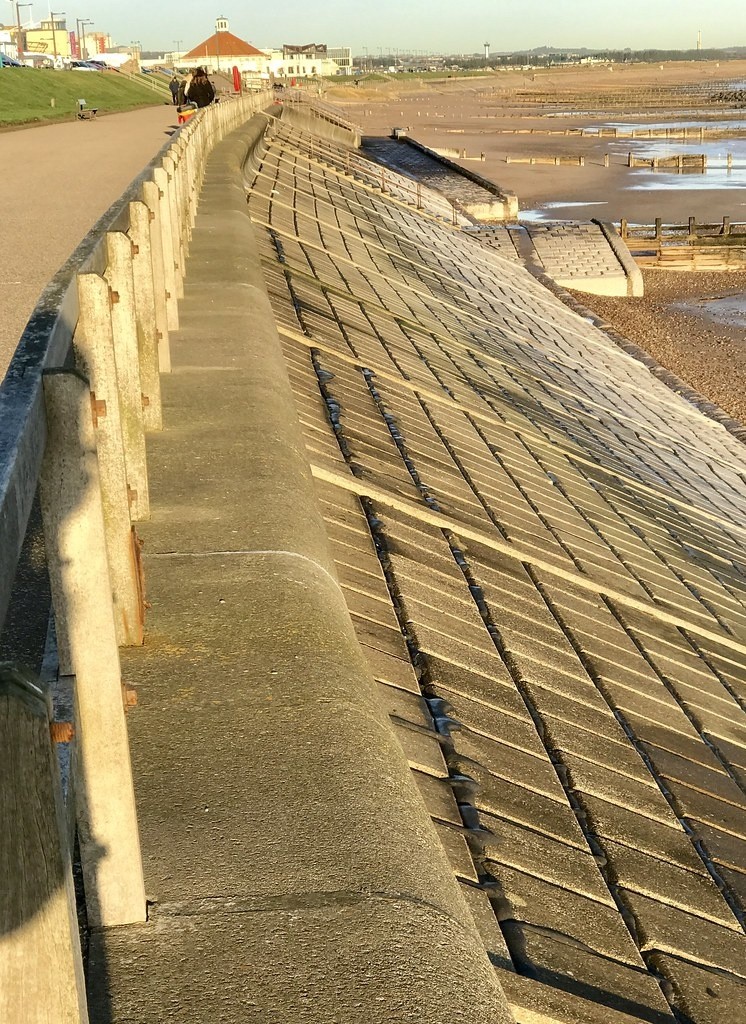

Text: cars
xmin=0 ymin=53 xmax=110 ymax=72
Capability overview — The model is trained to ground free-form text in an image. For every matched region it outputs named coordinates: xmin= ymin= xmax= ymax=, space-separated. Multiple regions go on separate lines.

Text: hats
xmin=194 ymin=70 xmax=208 ymax=78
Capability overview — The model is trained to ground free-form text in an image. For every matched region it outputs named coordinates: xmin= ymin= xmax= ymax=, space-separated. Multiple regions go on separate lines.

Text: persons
xmin=168 ymin=66 xmax=214 ymax=109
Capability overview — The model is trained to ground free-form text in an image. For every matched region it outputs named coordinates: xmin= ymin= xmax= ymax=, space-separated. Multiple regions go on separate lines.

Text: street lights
xmin=51 ymin=12 xmax=66 ymax=58
xmin=16 ymin=2 xmax=34 ymax=60
xmin=76 ymin=18 xmax=94 ymax=61
xmin=362 ymin=47 xmax=449 ymax=73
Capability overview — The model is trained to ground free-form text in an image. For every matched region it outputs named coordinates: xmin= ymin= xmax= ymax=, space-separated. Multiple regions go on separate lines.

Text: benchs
xmin=76 ymin=99 xmax=98 ymax=121
